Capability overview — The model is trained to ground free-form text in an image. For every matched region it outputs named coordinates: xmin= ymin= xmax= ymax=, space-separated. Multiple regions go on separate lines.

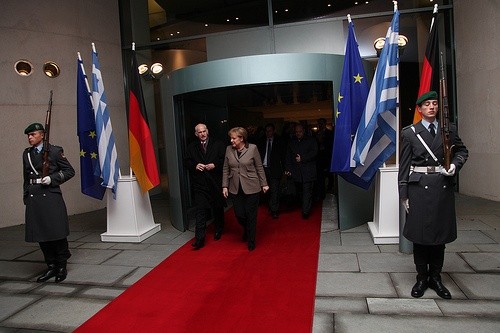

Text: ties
xmin=34 ymin=148 xmax=39 ymax=157
xmin=202 ymin=143 xmax=205 ymax=154
xmin=429 ymin=124 xmax=435 ymax=138
xmin=267 ymin=139 xmax=271 ymax=167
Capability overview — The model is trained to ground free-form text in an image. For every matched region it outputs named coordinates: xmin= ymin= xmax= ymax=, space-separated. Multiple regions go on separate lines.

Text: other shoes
xmin=192 ymin=242 xmax=204 ymax=248
xmin=214 ymin=232 xmax=222 ymax=240
xmin=302 ymin=211 xmax=309 ymax=219
xmin=272 ymin=212 xmax=278 ymax=218
xmin=241 ymin=234 xmax=248 ymax=242
xmin=248 ymin=242 xmax=255 ymax=251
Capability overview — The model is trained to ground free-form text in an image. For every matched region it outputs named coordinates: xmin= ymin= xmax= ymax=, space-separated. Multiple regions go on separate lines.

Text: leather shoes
xmin=37 ymin=264 xmax=56 ymax=282
xmin=429 ymin=278 xmax=451 ymax=299
xmin=411 ymin=279 xmax=428 ymax=298
xmin=55 ymin=262 xmax=66 ymax=283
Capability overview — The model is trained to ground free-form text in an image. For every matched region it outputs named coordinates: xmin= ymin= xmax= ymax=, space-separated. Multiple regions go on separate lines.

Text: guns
xmin=439 ymin=51 xmax=450 ymax=171
xmin=43 ymin=89 xmax=53 ymax=178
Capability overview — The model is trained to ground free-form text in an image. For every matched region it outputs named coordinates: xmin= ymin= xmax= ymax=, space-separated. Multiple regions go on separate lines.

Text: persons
xmin=398 ymin=91 xmax=470 ymax=301
xmin=249 ymin=118 xmax=333 ymax=218
xmin=222 ymin=127 xmax=269 ymax=250
xmin=23 ymin=123 xmax=75 ymax=283
xmin=185 ymin=123 xmax=225 ymax=247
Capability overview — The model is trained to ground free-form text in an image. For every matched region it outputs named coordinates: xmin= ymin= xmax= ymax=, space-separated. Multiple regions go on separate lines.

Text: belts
xmin=413 ymin=166 xmax=443 ymax=173
xmin=30 ymin=178 xmax=42 ymax=184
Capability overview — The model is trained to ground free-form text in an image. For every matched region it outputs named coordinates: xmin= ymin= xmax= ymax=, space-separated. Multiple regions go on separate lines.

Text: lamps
xmin=137 ymin=63 xmax=164 ymax=81
xmin=374 ymin=34 xmax=408 ymax=57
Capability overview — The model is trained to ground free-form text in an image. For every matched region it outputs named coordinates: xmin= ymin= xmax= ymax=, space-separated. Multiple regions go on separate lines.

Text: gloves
xmin=442 ymin=163 xmax=456 ymax=176
xmin=402 ymin=199 xmax=410 ymax=214
xmin=43 ymin=176 xmax=51 ymax=184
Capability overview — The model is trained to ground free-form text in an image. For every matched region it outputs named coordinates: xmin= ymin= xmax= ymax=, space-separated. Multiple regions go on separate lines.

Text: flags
xmin=331 ymin=20 xmax=374 ymax=190
xmin=413 ymin=13 xmax=441 ymax=123
xmin=350 ymin=10 xmax=400 ymax=182
xmin=91 ymin=50 xmax=119 ymax=200
xmin=76 ymin=59 xmax=107 ymax=201
xmin=129 ymin=92 xmax=160 ymax=194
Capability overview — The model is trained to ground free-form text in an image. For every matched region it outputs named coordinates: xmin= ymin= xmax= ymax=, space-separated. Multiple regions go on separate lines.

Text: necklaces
xmin=240 ymin=144 xmax=245 ymax=150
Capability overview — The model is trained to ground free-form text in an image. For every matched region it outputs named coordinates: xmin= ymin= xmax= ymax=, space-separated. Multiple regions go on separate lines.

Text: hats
xmin=24 ymin=123 xmax=44 ymax=134
xmin=416 ymin=91 xmax=438 ymax=105
